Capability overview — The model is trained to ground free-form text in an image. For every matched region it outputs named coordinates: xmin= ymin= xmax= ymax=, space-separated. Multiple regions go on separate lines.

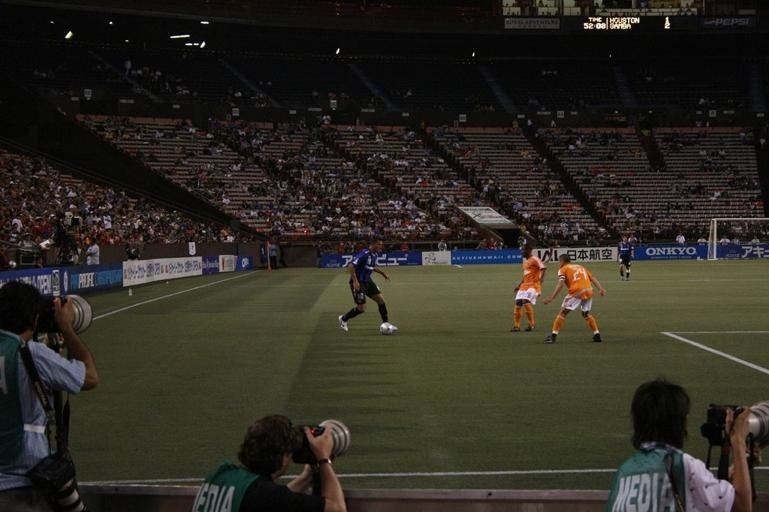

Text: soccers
xmin=379 ymin=322 xmax=393 ymax=334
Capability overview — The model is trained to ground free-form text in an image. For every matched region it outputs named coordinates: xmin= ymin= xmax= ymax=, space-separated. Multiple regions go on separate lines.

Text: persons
xmin=0 ymin=130 xmax=281 ymax=281
xmin=191 ymin=408 xmax=349 ymax=512
xmin=504 ymin=243 xmax=547 ymax=333
xmin=541 ymin=254 xmax=606 ymax=346
xmin=334 ymin=234 xmax=402 ymax=336
xmin=615 ymin=235 xmax=634 ymax=282
xmin=606 ymin=376 xmax=763 ymax=512
xmin=1 ymin=277 xmax=102 ymax=511
xmin=37 ymin=43 xmax=768 ymax=248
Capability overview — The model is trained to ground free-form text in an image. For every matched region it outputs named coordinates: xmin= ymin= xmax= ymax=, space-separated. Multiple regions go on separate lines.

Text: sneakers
xmin=511 ymin=326 xmax=535 ymax=332
xmin=338 ymin=315 xmax=349 ymax=332
xmin=592 ymin=334 xmax=601 ymax=341
xmin=545 ymin=336 xmax=555 ymax=342
xmin=392 ymin=325 xmax=398 ymax=331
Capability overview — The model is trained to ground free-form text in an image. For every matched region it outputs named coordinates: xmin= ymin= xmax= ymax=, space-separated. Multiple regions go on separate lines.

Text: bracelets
xmin=316 ymin=458 xmax=333 ymax=471
